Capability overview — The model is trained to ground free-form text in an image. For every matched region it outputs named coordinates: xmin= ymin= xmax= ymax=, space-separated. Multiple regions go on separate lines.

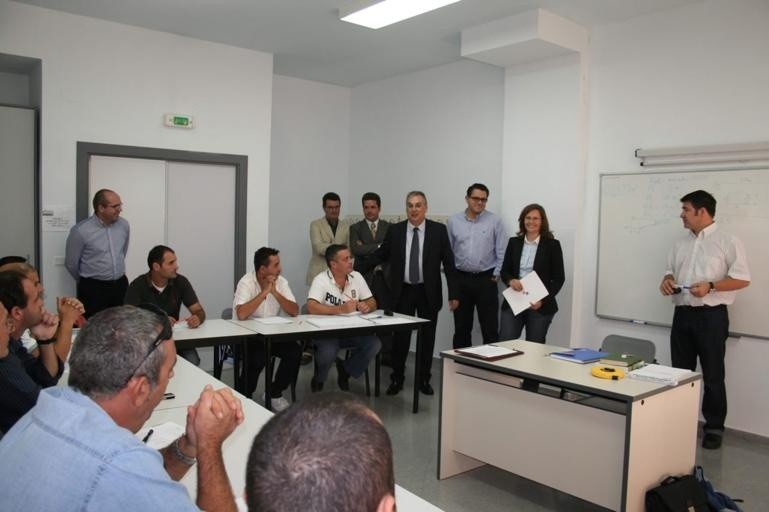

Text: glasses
xmin=470 ymin=196 xmax=487 ymax=202
xmin=108 ymin=204 xmax=120 ymax=208
xmin=125 ymin=302 xmax=172 ymax=385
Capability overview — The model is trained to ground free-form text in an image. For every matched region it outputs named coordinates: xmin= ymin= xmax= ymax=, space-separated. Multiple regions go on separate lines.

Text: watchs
xmin=707 ymin=281 xmax=717 ymax=294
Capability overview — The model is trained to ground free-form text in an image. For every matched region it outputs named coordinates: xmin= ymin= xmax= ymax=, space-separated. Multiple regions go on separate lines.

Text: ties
xmin=371 ymin=223 xmax=377 ymax=241
xmin=409 ymin=227 xmax=420 ymax=282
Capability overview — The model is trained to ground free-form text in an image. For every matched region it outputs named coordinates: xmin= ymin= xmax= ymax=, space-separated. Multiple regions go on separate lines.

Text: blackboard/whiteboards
xmin=595 ymin=164 xmax=769 ymax=340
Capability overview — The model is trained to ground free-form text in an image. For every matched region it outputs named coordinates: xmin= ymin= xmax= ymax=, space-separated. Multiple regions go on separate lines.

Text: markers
xmin=628 ymin=320 xmax=648 ymax=325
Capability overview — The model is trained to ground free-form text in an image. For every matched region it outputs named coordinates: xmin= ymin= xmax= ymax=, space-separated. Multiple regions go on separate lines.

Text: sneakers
xmin=703 ymin=433 xmax=722 ymax=449
xmin=263 ymin=392 xmax=289 ymax=412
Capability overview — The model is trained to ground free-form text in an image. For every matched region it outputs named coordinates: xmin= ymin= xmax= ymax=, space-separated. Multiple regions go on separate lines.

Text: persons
xmin=444 ymin=182 xmax=509 ymax=349
xmin=657 ymin=189 xmax=753 ymax=450
xmin=64 ymin=189 xmax=397 ymax=412
xmin=497 ymin=204 xmax=566 ymax=345
xmin=366 ymin=189 xmax=461 ymax=396
xmin=1 ymin=255 xmax=395 ymax=511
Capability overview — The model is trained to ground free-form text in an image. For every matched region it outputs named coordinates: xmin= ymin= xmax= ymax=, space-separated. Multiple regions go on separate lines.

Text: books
xmin=548 ymin=346 xmax=612 ymax=365
xmin=598 ymin=351 xmax=645 ymax=373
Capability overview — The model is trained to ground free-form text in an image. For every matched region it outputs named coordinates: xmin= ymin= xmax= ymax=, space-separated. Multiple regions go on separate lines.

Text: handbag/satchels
xmin=702 ymin=478 xmax=726 ymax=509
xmin=644 ymin=474 xmax=713 ymax=512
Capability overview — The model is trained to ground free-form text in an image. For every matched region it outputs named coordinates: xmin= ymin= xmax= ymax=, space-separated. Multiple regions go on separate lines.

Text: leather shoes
xmin=336 ymin=363 xmax=350 ymax=392
xmin=311 ymin=375 xmax=324 ymax=392
xmin=420 ymin=383 xmax=434 ymax=395
xmin=386 ymin=380 xmax=403 ymax=395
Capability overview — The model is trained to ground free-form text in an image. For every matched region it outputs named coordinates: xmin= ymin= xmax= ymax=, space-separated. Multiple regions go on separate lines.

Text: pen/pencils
xmin=344 ymin=293 xmax=354 ymax=301
xmin=678 ymin=286 xmax=692 ymax=289
xmin=142 ymin=429 xmax=154 ymax=443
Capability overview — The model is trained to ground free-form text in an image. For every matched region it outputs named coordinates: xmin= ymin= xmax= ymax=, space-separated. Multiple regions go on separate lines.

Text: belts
xmin=462 ymin=270 xmax=489 ymax=279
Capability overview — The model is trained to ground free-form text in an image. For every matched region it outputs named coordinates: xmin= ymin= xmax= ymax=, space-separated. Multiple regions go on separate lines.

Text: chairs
xmin=301 ymin=304 xmax=370 ymax=399
xmin=599 ymin=334 xmax=657 ymax=364
xmin=218 ymin=308 xmax=295 ymax=402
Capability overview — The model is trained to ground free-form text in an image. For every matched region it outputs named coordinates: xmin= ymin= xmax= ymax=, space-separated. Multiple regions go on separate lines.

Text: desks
xmin=132 ymin=399 xmax=442 ymax=512
xmin=56 ymin=354 xmax=248 ymax=410
xmin=437 ymin=338 xmax=704 ymax=512
xmin=226 ymin=309 xmax=431 ymax=414
xmin=172 ymin=319 xmax=260 ymax=391
